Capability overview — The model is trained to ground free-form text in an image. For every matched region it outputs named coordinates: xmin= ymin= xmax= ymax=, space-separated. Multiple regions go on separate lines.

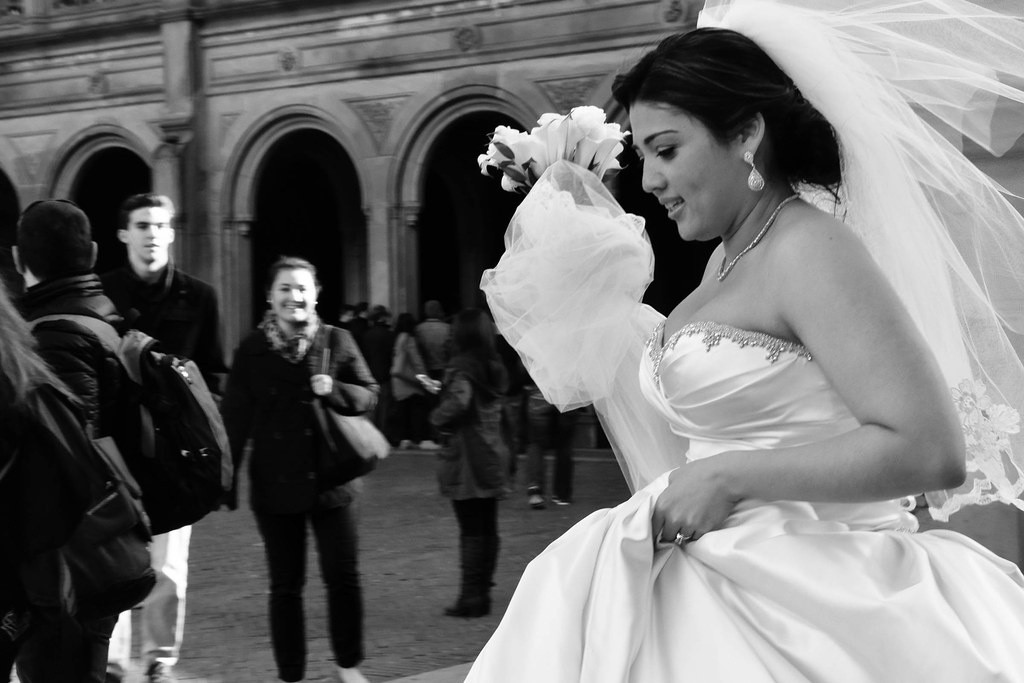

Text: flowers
xmin=474 ymin=103 xmax=633 ymax=205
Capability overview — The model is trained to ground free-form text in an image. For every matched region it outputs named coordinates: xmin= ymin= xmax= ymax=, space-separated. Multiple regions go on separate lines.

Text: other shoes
xmin=420 ymin=441 xmax=442 ymax=450
xmin=337 ymin=666 xmax=369 ymax=683
xmin=552 ymin=496 xmax=571 ymax=506
xmin=529 ymin=494 xmax=544 ymax=507
xmin=399 ymin=440 xmax=417 ymax=450
xmin=444 ymin=604 xmax=490 ymax=618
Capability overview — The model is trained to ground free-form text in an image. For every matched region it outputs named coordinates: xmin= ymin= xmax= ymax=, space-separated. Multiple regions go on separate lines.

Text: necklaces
xmin=717 ymin=193 xmax=799 ymax=281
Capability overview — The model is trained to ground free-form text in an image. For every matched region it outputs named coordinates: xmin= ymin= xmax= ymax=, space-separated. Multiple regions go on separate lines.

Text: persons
xmin=473 ymin=0 xmax=1024 ymax=683
xmin=0 ymin=190 xmax=575 ymax=683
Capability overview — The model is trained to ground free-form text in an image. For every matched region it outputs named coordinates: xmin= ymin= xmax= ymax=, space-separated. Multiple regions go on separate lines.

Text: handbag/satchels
xmin=390 ymin=349 xmax=420 ymax=383
xmin=312 ymin=326 xmax=392 ymax=482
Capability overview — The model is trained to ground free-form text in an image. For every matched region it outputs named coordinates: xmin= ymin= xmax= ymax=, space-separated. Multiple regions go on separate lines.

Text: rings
xmin=675 ymin=532 xmax=693 ymax=545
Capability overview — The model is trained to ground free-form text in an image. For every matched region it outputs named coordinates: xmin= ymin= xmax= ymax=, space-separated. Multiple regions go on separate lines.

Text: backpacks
xmin=0 ymin=379 xmax=157 ymax=618
xmin=25 ymin=314 xmax=239 ymax=535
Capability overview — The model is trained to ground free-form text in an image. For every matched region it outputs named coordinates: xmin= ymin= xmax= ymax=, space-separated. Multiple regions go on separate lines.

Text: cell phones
xmin=416 ymin=373 xmax=443 ymax=390
